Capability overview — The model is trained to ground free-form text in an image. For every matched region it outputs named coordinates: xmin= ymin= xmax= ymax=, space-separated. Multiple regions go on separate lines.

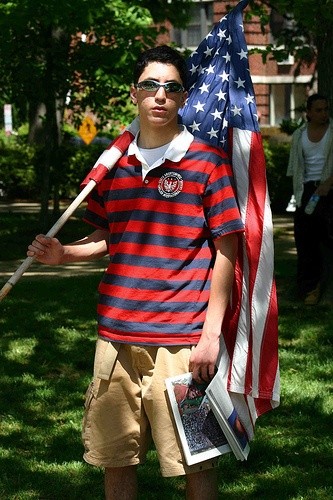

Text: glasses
xmin=134 ymin=80 xmax=185 ymax=95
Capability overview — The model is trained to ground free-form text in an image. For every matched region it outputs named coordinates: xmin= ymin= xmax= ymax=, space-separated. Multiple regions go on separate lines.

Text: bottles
xmin=305 ymin=193 xmax=320 ymax=214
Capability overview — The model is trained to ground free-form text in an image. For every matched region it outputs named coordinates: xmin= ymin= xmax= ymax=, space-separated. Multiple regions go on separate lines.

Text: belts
xmin=304 ymin=180 xmax=322 ymax=187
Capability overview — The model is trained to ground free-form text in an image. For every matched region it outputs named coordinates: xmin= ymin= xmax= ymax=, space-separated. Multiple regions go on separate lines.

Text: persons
xmin=286 ymin=93 xmax=333 ymax=304
xmin=27 ymin=45 xmax=244 ymax=500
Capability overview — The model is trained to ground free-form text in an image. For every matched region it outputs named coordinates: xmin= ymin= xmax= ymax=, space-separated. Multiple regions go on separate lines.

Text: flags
xmin=79 ymin=2 xmax=282 ymax=427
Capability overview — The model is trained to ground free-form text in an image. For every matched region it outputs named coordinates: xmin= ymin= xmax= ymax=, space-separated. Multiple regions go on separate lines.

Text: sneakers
xmin=304 ymin=280 xmax=321 ymax=306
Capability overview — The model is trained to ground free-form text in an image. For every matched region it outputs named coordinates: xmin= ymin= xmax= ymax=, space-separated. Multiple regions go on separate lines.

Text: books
xmin=164 ymin=364 xmax=252 ymax=466
